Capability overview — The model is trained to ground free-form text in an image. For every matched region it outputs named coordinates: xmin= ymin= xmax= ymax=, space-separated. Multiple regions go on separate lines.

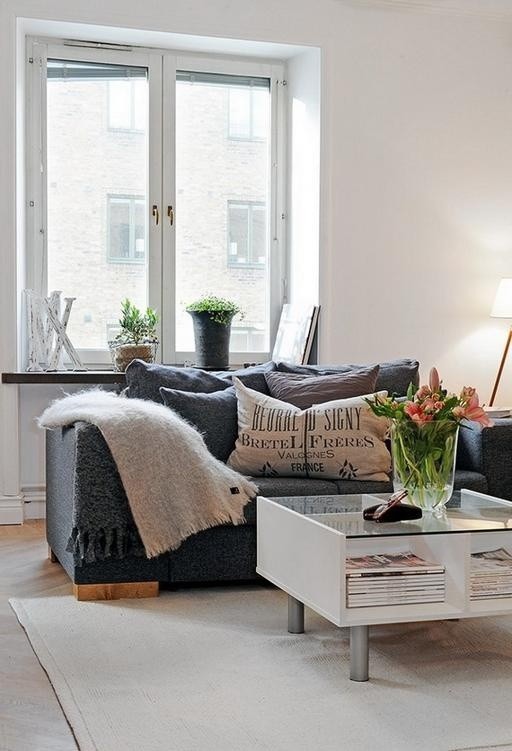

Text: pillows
xmin=279 ymin=354 xmax=421 ymax=402
xmin=261 ymin=363 xmax=383 ymax=411
xmin=158 ymin=386 xmax=237 ymax=466
xmin=226 ymin=376 xmax=389 ymax=484
xmin=123 ymin=358 xmax=277 ymax=403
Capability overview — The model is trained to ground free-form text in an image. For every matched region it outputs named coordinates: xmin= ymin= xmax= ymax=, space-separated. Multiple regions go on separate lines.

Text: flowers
xmin=361 ymin=367 xmax=495 ymax=507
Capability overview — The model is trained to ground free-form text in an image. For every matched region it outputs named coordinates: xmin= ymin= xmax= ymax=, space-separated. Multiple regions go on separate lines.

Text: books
xmin=469 ymin=548 xmax=512 ymax=602
xmin=271 ymin=303 xmax=321 ymax=366
xmin=345 ymin=547 xmax=447 ymax=608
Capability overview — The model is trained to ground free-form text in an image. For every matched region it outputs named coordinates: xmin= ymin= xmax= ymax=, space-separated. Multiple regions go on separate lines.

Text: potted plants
xmin=185 ymin=295 xmax=245 ymax=371
xmin=107 ymin=298 xmax=161 ymax=372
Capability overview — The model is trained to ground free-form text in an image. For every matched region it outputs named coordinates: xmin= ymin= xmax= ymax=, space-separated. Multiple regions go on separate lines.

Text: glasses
xmin=365 ymin=489 xmax=408 ymax=522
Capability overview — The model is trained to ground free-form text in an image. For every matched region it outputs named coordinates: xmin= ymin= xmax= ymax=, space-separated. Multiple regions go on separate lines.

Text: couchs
xmin=46 ymin=357 xmax=512 ymax=601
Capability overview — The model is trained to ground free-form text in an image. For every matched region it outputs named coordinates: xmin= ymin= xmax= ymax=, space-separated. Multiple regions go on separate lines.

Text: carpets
xmin=7 ymin=585 xmax=510 ymax=750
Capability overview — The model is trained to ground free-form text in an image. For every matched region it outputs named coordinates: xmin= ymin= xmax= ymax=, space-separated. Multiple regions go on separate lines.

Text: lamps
xmin=483 ymin=277 xmax=512 ymax=418
